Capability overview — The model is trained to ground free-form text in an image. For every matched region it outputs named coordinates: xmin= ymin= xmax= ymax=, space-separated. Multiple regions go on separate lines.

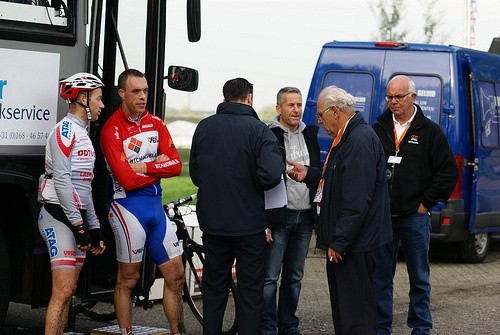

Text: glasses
xmin=384 ymin=92 xmax=411 ymax=102
xmin=315 ymin=106 xmax=330 ymax=119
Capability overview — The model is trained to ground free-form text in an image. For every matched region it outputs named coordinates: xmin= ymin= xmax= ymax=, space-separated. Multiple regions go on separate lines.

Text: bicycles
xmin=76 ymin=192 xmax=239 ymax=335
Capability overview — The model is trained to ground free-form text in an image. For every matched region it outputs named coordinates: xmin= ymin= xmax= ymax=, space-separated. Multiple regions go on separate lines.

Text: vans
xmin=300 ymin=40 xmax=500 ymax=263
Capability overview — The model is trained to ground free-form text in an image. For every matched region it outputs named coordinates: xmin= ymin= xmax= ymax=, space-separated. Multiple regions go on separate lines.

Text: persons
xmin=285 ymin=84 xmax=392 ymax=335
xmin=188 ymin=78 xmax=285 ymax=335
xmin=37 ymin=72 xmax=107 ymax=335
xmin=263 ymin=87 xmax=322 ymax=335
xmin=371 ymin=74 xmax=460 ymax=335
xmin=100 ymin=69 xmax=186 ymax=335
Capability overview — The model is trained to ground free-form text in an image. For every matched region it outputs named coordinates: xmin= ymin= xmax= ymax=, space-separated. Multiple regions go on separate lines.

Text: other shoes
xmin=288 ymin=332 xmax=301 ymax=335
xmin=128 ymin=331 xmax=135 ymax=335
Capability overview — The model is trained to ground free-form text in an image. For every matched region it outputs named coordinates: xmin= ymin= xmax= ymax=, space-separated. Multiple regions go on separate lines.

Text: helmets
xmin=58 ymin=72 xmax=106 ymax=121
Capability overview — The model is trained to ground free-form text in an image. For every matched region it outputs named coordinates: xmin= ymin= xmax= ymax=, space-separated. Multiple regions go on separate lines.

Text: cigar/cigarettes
xmin=329 ymin=256 xmax=333 ymax=261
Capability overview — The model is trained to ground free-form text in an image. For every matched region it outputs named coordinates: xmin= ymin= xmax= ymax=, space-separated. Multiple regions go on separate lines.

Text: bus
xmin=0 ymin=0 xmax=202 ymax=322
